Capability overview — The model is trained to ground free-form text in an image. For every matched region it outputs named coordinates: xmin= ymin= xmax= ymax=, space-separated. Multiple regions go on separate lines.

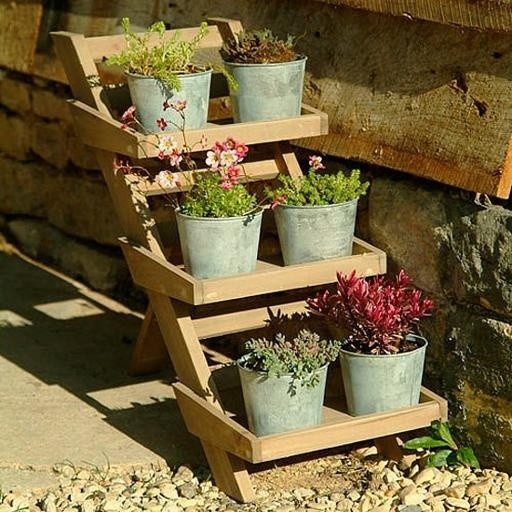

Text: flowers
xmin=112 ymin=96 xmax=372 ymax=217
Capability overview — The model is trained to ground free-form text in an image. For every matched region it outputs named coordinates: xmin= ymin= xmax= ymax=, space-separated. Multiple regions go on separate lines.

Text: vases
xmin=173 ymin=198 xmax=359 ymax=282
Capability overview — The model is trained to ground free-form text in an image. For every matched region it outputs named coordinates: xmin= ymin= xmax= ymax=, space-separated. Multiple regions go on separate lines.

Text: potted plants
xmin=234 ymin=267 xmax=437 ymax=436
xmin=105 ymin=16 xmax=308 ymax=136
